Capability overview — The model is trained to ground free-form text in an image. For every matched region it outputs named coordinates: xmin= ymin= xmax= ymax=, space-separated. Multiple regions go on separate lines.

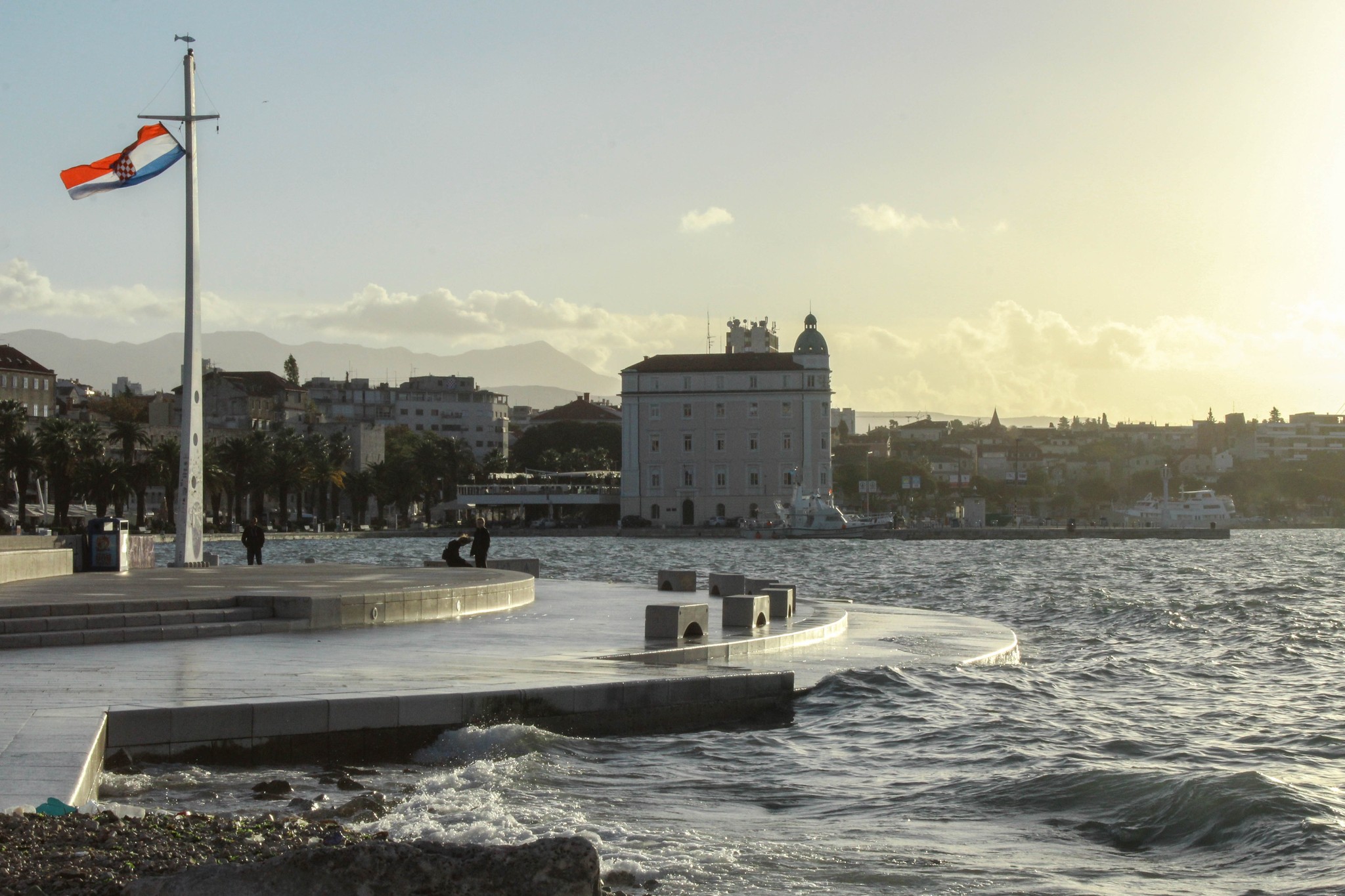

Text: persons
xmin=242 ymin=518 xmax=266 ymax=566
xmin=39 ymin=520 xmax=44 ymax=527
xmin=447 ymin=535 xmax=473 ymax=567
xmin=469 ymin=517 xmax=491 ymax=569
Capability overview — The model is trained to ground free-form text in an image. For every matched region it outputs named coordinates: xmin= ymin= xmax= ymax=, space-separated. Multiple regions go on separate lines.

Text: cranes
xmin=904 ymin=411 xmax=925 ymax=425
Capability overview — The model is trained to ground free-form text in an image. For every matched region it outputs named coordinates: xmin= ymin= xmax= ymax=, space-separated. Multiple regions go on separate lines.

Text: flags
xmin=59 ymin=120 xmax=186 ymax=201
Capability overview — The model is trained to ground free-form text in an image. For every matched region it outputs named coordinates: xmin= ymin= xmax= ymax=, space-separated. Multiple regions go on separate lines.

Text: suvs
xmin=560 ymin=517 xmax=587 ymax=529
xmin=621 ymin=515 xmax=652 ymax=528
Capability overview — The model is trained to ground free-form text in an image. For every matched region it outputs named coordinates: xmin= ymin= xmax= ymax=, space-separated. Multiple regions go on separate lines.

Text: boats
xmin=1125 ymin=483 xmax=1236 ymax=527
xmin=773 ymin=480 xmax=897 ymax=530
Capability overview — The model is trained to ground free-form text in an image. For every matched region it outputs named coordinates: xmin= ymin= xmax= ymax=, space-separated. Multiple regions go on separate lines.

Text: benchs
xmin=761 ymin=588 xmax=793 ymax=618
xmin=645 ymin=602 xmax=709 ymax=640
xmin=745 ymin=578 xmax=779 ymax=594
xmin=722 ymin=594 xmax=770 ymax=628
xmin=769 ymin=583 xmax=796 ymax=612
xmin=709 ymin=572 xmax=745 ymax=595
xmin=658 ymin=569 xmax=696 ymax=592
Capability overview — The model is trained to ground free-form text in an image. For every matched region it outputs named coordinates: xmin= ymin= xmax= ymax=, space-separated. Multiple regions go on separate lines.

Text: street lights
xmin=866 ymin=450 xmax=873 ymax=513
xmin=437 ymin=477 xmax=445 ymax=527
xmin=1015 ymin=438 xmax=1023 ymax=519
xmin=1160 ymin=463 xmax=1172 ymax=527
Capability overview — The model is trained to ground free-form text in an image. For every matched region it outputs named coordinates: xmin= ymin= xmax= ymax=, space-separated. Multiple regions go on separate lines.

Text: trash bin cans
xmin=87 ymin=517 xmax=129 ymax=571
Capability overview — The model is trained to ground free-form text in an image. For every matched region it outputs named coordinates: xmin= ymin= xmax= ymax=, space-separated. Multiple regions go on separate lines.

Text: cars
xmin=708 ymin=516 xmax=732 ymax=527
xmin=532 ymin=518 xmax=560 ymax=528
xmin=1249 ymin=516 xmax=1270 ymax=522
